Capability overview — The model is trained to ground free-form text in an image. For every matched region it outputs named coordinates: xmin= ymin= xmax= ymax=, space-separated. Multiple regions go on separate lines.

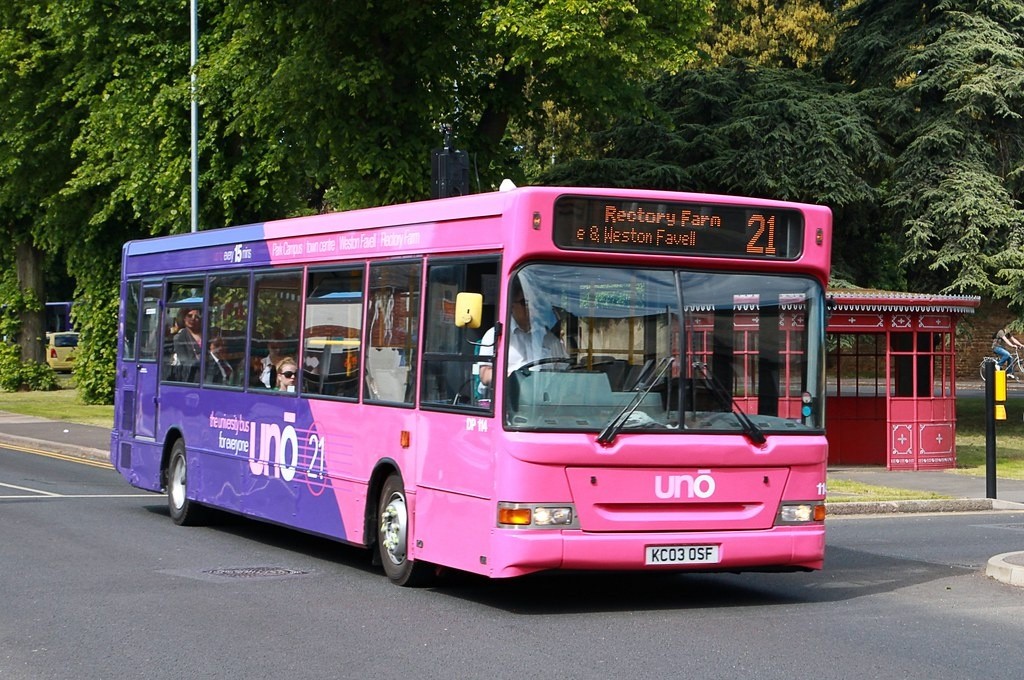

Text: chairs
xmin=470 ymin=340 xmax=486 ymax=407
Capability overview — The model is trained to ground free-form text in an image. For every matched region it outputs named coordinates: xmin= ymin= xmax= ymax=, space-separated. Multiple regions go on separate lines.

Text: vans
xmin=45 ymin=330 xmax=81 ymax=374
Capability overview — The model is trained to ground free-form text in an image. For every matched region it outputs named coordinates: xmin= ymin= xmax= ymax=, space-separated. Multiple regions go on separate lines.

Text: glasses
xmin=279 ymin=371 xmax=298 ymax=378
xmin=185 ymin=314 xmax=200 ymax=318
xmin=513 ymin=299 xmax=529 ymax=308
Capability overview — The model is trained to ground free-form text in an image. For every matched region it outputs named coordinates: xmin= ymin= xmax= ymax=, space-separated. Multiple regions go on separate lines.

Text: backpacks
xmin=991 ymin=328 xmax=1006 ymax=340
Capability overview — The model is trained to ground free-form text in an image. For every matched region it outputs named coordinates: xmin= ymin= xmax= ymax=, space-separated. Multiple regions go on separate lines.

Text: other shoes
xmin=994 ymin=363 xmax=1002 ymax=372
xmin=1006 ymin=373 xmax=1015 ymax=379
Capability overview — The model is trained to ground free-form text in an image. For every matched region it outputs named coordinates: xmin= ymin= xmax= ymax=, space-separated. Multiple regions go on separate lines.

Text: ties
xmin=270 ymin=366 xmax=277 ymax=388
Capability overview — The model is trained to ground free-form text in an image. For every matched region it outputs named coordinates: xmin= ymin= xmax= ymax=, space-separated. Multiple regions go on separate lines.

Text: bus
xmin=110 ymin=176 xmax=834 ymax=587
xmin=45 ymin=302 xmax=80 ymax=332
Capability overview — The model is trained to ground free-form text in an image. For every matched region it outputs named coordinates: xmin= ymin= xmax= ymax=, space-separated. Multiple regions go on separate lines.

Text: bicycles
xmin=978 ymin=345 xmax=1024 ymax=384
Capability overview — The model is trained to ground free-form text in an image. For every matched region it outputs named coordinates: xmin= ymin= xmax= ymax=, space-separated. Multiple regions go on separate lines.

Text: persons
xmin=992 ymin=323 xmax=1024 ymax=378
xmin=172 ymin=305 xmax=298 ymax=391
xmin=479 ymin=290 xmax=569 ymax=399
